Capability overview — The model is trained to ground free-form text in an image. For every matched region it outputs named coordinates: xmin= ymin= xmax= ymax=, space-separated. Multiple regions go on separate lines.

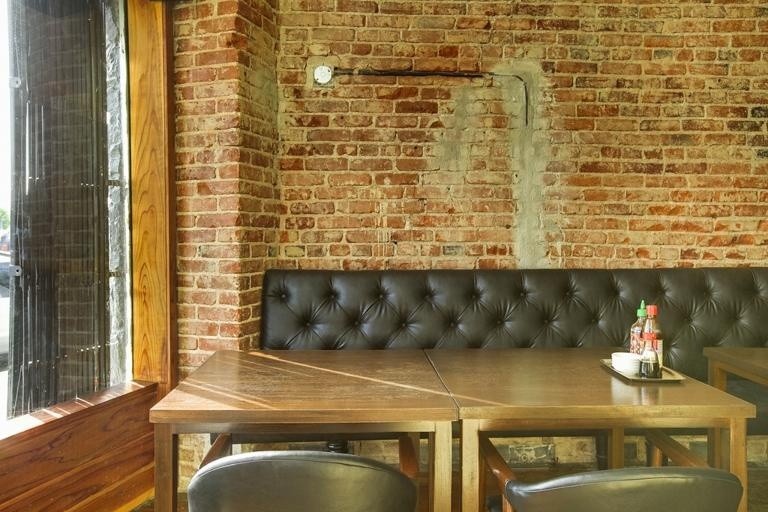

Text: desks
xmin=702 ymin=344 xmax=768 ymax=472
xmin=425 ymin=344 xmax=757 ymax=512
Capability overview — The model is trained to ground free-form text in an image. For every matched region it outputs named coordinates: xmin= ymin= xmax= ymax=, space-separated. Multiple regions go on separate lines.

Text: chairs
xmin=474 ymin=431 xmax=743 ymax=512
xmin=187 ymin=433 xmax=421 ymax=512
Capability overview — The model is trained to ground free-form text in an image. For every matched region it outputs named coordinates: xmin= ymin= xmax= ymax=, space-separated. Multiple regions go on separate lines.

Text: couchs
xmin=258 ymin=266 xmax=764 ymax=436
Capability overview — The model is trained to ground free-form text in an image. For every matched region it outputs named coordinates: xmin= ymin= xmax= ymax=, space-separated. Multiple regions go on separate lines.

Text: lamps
xmin=314 ymin=65 xmax=529 ymax=127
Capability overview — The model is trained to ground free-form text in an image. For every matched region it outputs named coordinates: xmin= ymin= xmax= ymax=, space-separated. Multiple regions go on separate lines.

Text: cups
xmin=612 ymin=351 xmax=640 ymax=375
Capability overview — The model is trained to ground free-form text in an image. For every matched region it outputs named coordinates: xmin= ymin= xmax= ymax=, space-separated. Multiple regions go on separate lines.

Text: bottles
xmin=631 ymin=299 xmax=663 ymax=377
